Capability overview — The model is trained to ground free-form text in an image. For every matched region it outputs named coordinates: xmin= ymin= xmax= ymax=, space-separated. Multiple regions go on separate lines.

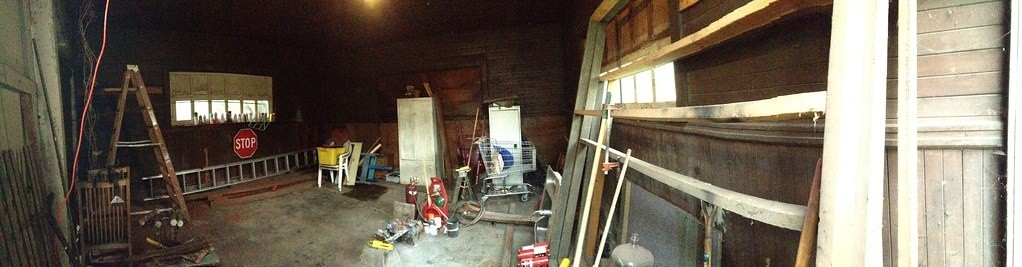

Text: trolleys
xmin=472 ymin=137 xmax=537 ymax=203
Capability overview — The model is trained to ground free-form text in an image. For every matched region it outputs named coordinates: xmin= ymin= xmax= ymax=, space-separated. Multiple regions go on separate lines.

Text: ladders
xmin=141 ymin=145 xmax=331 ymax=202
xmin=103 ymin=64 xmax=191 ymax=224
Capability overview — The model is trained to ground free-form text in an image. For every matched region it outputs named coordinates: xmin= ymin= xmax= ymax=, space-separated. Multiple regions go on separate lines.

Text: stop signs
xmin=232 ymin=130 xmax=257 ymax=159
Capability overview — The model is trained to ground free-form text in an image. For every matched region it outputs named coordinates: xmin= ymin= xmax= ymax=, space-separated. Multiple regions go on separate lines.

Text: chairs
xmin=317 ymin=140 xmax=352 ymax=191
xmin=356 ymin=152 xmax=392 ymax=184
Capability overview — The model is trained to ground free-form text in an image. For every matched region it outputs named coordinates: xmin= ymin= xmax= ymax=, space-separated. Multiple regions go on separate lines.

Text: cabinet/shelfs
xmin=397 ymin=98 xmax=446 ymax=186
xmin=488 ymin=105 xmax=523 ymax=187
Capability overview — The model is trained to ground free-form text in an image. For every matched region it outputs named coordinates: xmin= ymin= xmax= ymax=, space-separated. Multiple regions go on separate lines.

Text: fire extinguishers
xmin=406 ymin=177 xmax=420 ymax=220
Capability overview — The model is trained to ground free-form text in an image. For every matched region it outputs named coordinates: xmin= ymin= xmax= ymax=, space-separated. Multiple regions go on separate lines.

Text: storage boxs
xmin=317 ymin=145 xmax=348 ymax=164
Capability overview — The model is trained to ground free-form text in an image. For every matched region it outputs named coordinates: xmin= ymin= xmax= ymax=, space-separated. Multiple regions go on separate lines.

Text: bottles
xmin=199 ymin=115 xmax=202 ymax=124
xmin=210 ymin=113 xmax=213 ymax=123
xmin=221 ymin=113 xmax=224 ymax=123
xmin=234 ymin=114 xmax=237 ymax=122
xmin=259 ymin=113 xmax=267 ymax=121
xmin=203 ymin=115 xmax=206 ymax=124
xmin=249 ymin=112 xmax=253 ymax=122
xmin=255 ymin=113 xmax=258 ymax=122
xmin=193 ymin=112 xmax=198 ymax=125
xmin=269 ymin=113 xmax=275 ymax=122
xmin=226 ymin=111 xmax=231 ymax=122
xmin=238 ymin=114 xmax=241 ymax=122
xmin=214 ymin=112 xmax=217 ymax=123
xmin=296 ymin=105 xmax=302 ymax=121
xmin=243 ymin=113 xmax=248 ymax=122
